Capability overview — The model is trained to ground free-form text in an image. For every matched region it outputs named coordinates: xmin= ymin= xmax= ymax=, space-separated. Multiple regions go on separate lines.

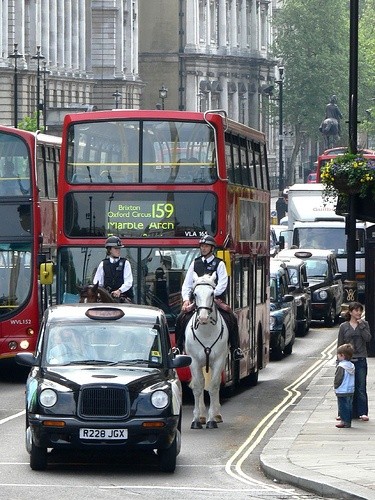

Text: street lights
xmin=9 ymin=43 xmax=22 ymax=128
xmin=112 ymin=90 xmax=120 ymax=108
xmin=40 ymin=62 xmax=50 ymax=128
xmin=159 ymin=84 xmax=167 ymax=109
xmin=31 ymin=46 xmax=44 ymax=129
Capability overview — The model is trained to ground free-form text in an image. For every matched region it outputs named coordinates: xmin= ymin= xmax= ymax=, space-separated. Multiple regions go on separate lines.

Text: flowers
xmin=321 ymin=153 xmax=375 ymax=198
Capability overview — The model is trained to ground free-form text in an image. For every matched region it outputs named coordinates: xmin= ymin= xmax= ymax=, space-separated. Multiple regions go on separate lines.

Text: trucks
xmin=271 ymin=217 xmax=367 ymax=302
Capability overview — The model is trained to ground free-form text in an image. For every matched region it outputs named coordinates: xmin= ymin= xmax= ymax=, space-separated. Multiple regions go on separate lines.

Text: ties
xmin=204 ymin=259 xmax=207 ymax=264
xmin=113 ymin=259 xmax=116 ymax=263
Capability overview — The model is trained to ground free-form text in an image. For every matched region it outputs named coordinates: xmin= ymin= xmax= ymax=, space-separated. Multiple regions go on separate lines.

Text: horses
xmin=184 ymin=270 xmax=230 ymax=430
xmin=73 ymin=281 xmax=132 ymax=303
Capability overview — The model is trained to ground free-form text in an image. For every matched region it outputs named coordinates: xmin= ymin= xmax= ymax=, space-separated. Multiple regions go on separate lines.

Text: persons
xmin=2 ymin=161 xmax=18 ymax=185
xmin=171 ymin=236 xmax=243 ymax=362
xmin=124 ymin=331 xmax=180 ymax=365
xmin=334 ymin=344 xmax=354 ymax=428
xmin=299 ymin=228 xmax=319 ymax=249
xmin=148 ymin=267 xmax=169 ymax=309
xmin=326 ymin=95 xmax=343 ymax=135
xmin=89 ymin=236 xmax=134 ymax=303
xmin=48 ymin=329 xmax=98 ymax=365
xmin=336 ymin=301 xmax=372 ymax=422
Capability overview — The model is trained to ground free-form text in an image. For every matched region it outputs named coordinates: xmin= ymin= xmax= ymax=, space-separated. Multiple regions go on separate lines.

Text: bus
xmin=0 ymin=125 xmax=61 ymax=374
xmin=56 ymin=108 xmax=269 ymax=391
xmin=316 ymin=146 xmax=375 ymax=183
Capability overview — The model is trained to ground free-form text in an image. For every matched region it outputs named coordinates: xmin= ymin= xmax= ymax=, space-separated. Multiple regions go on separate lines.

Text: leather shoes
xmin=361 ymin=416 xmax=369 ymax=422
xmin=173 ymin=348 xmax=182 ymax=356
xmin=231 ymin=351 xmax=244 ymax=360
xmin=336 ymin=417 xmax=341 ymax=420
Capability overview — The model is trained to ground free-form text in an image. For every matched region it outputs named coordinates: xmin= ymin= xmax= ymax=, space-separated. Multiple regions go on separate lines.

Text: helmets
xmin=105 ymin=235 xmax=125 ymax=248
xmin=199 ymin=235 xmax=216 ymax=246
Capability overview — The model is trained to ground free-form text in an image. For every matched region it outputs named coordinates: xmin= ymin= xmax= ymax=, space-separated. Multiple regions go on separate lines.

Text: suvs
xmin=15 ymin=302 xmax=192 ymax=472
xmin=269 ymin=261 xmax=297 ymax=358
xmin=286 ymin=258 xmax=311 ymax=337
xmin=278 ymin=248 xmax=343 ymax=327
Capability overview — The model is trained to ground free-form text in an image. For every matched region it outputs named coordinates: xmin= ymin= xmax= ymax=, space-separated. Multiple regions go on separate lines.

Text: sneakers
xmin=335 ymin=422 xmax=351 ymax=428
